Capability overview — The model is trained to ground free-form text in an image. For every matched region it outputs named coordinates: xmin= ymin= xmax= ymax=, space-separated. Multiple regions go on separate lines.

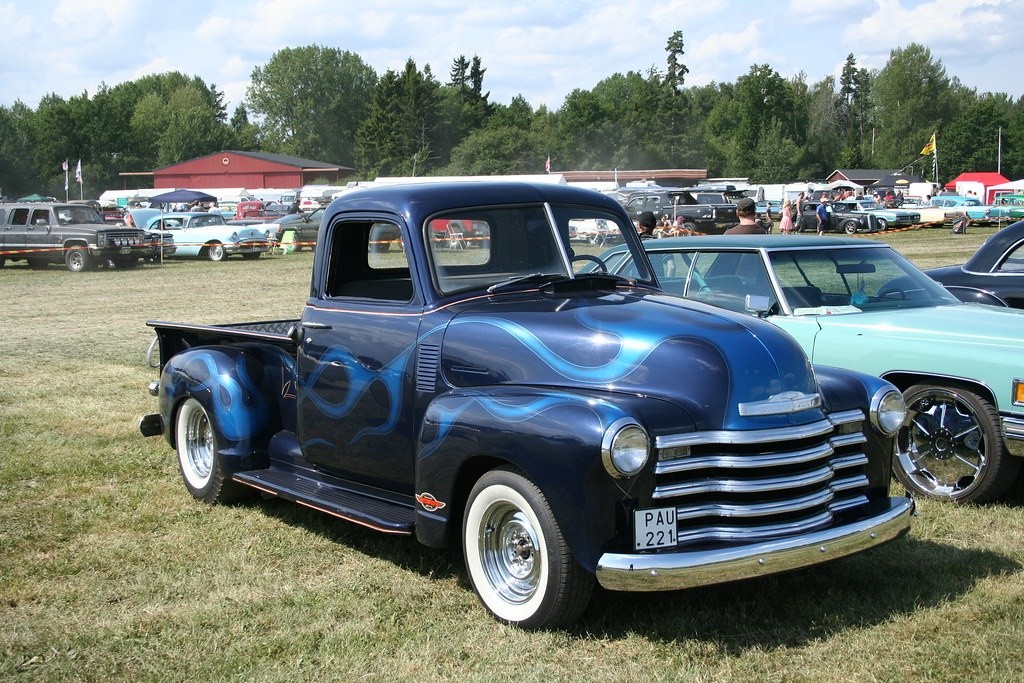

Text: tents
xmin=826 ymin=173 xmax=1024 ymax=206
xmin=148 ymin=189 xmax=217 ymax=203
xmin=17 ymin=193 xmax=54 ymax=203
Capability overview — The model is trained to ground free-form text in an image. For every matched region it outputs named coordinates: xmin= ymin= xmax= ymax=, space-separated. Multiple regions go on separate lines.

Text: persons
xmin=723 ymin=198 xmax=768 ymax=235
xmin=765 ymin=202 xmax=774 ymax=234
xmin=780 ymin=186 xmax=943 ymax=237
xmin=656 ymin=213 xmax=686 ymax=229
xmin=629 ymin=210 xmax=677 ymax=279
xmin=191 ymin=200 xmax=222 ymax=214
xmin=754 ymin=213 xmax=765 ymax=228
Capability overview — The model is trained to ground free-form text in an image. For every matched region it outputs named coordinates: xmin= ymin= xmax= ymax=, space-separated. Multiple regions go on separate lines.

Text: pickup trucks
xmin=138 ymin=177 xmax=916 ymax=632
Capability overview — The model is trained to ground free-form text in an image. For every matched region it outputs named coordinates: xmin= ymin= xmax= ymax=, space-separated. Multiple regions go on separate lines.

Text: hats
xmin=637 ymin=211 xmax=656 ymax=229
xmin=676 ymin=215 xmax=685 ymax=221
xmin=821 ymin=197 xmax=829 ymax=203
xmin=737 ymin=198 xmax=757 ymax=211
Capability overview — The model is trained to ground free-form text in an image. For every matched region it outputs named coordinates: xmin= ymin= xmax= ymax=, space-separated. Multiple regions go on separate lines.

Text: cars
xmin=875 ymin=219 xmax=1024 ymax=308
xmin=1 ymin=201 xmax=153 ymax=273
xmin=574 ymin=233 xmax=1024 ymax=508
xmin=66 ymin=176 xmax=1023 ymax=257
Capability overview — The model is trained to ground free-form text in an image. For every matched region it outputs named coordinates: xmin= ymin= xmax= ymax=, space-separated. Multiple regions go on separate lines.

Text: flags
xmin=545 ymin=156 xmax=550 ymax=172
xmin=920 ymin=133 xmax=935 ymax=156
xmin=76 ymin=159 xmax=83 ymax=183
xmin=61 ymin=162 xmax=69 ymax=191
